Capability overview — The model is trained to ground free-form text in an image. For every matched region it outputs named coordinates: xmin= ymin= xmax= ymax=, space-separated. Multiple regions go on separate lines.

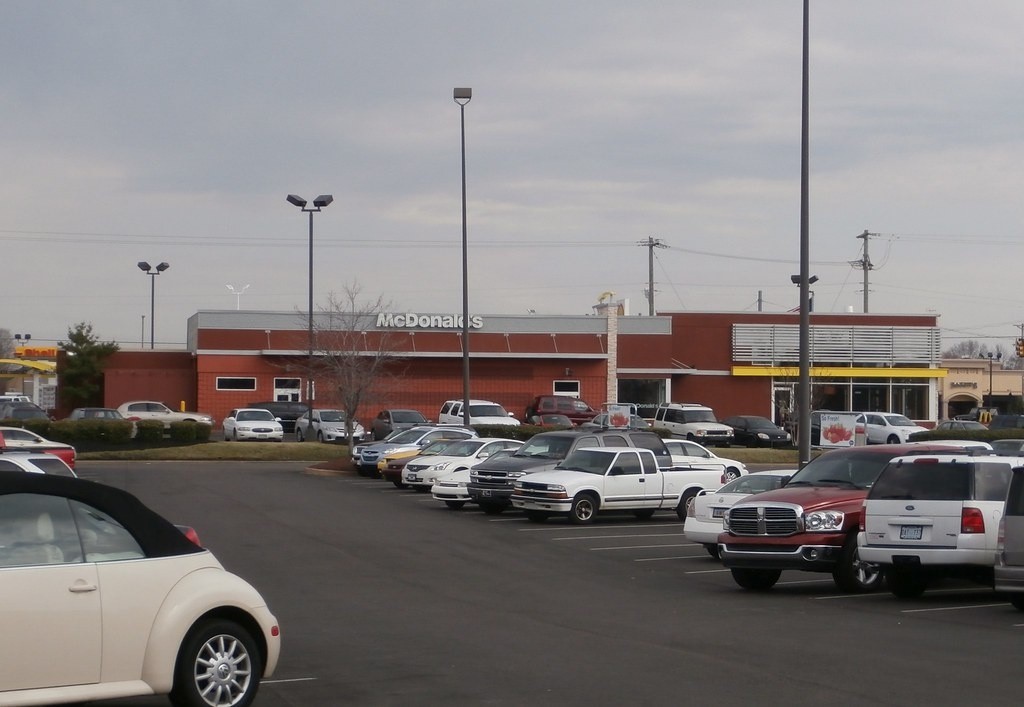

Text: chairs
xmin=34 ymin=512 xmax=63 ymax=563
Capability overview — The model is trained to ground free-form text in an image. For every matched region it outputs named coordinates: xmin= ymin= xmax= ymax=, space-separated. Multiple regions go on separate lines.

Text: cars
xmin=95 ymin=401 xmax=216 ymax=427
xmin=0 ymin=452 xmax=77 ymax=479
xmin=294 ymin=409 xmax=365 ymax=443
xmin=350 ymin=395 xmax=1024 ymax=611
xmin=0 ymin=400 xmax=52 ymax=420
xmin=0 ymin=426 xmax=77 ymax=469
xmin=246 ymin=402 xmax=313 ymax=433
xmin=71 ymin=408 xmax=125 ymax=420
xmin=222 ymin=408 xmax=284 ymax=442
xmin=0 ymin=397 xmax=30 ymax=402
xmin=0 ymin=471 xmax=281 ymax=707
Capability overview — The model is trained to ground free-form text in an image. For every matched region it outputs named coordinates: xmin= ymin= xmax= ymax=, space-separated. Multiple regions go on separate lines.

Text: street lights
xmin=979 ymin=353 xmax=1002 ymax=394
xmin=141 ymin=315 xmax=146 ymax=348
xmin=286 ymin=194 xmax=334 ymax=443
xmin=137 ymin=262 xmax=169 ymax=349
xmin=453 ymin=88 xmax=472 ymax=427
xmin=14 ymin=334 xmax=31 ymax=396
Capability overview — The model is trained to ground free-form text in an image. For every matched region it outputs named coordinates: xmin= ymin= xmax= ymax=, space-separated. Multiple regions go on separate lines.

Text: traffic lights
xmin=1016 ymin=340 xmax=1024 ymax=358
